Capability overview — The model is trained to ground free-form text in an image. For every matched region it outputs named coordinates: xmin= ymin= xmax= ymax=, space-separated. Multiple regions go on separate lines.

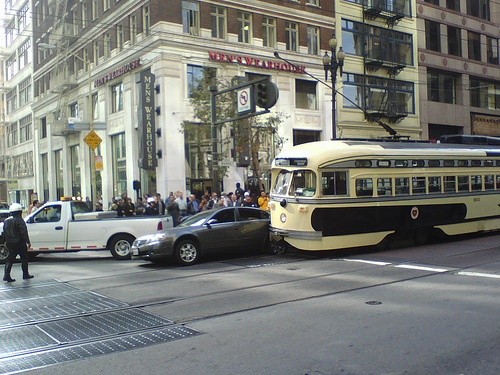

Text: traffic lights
xmin=253 ymin=80 xmax=278 ymax=108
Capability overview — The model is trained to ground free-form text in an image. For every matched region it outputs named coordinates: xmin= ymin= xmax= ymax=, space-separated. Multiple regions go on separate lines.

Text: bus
xmin=270 ymin=140 xmax=500 ymax=251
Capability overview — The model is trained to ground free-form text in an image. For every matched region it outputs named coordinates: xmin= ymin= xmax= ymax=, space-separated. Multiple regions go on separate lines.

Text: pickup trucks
xmin=0 ymin=200 xmax=174 ymax=264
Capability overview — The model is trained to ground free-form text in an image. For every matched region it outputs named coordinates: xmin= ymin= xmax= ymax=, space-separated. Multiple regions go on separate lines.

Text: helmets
xmin=147 ymin=198 xmax=155 ymax=203
xmin=9 ymin=203 xmax=22 ymax=211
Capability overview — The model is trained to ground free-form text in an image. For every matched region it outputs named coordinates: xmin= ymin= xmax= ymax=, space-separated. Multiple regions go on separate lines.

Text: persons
xmin=32 ymin=199 xmax=39 ymax=212
xmin=1 ymin=202 xmax=33 ymax=282
xmin=85 ymin=198 xmax=92 ymax=211
xmin=258 ymin=190 xmax=270 ymax=208
xmin=109 ymin=182 xmax=256 ymax=217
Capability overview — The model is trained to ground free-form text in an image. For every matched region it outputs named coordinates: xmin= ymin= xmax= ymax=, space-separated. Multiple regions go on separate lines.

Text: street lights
xmin=323 ymin=34 xmax=344 ymax=137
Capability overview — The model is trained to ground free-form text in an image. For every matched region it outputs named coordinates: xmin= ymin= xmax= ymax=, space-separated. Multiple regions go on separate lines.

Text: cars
xmin=132 ymin=207 xmax=286 ymax=265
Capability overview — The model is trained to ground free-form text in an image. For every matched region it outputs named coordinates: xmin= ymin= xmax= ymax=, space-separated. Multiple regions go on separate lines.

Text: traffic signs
xmin=83 ymin=129 xmax=102 ymax=150
xmin=94 ymin=155 xmax=103 ymax=170
xmin=238 ymin=88 xmax=251 ymax=112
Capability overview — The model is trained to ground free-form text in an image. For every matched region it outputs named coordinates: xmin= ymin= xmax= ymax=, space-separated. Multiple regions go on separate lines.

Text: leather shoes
xmin=2 ymin=263 xmax=15 ymax=282
xmin=21 ymin=261 xmax=33 ymax=279
